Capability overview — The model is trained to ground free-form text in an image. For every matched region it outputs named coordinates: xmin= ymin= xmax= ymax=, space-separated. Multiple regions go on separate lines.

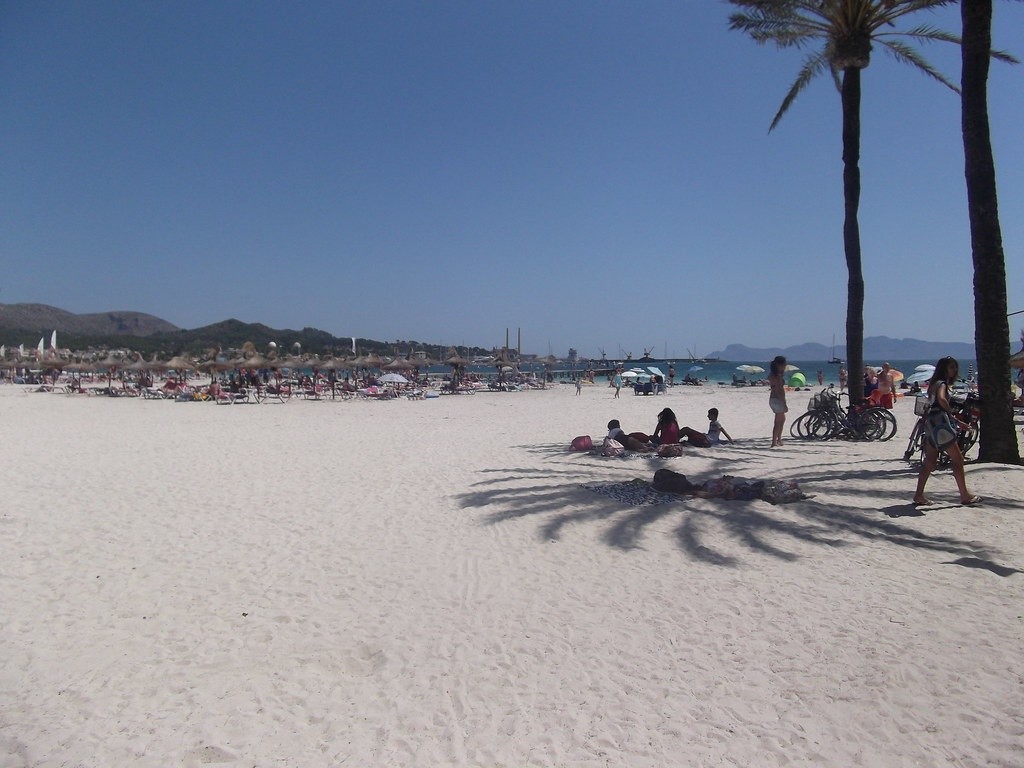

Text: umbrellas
xmin=785 ymin=364 xmax=800 ymax=371
xmin=736 ymin=365 xmax=766 ymax=375
xmin=907 ymin=364 xmax=936 ymax=383
xmin=690 ymin=366 xmax=704 ymax=372
xmin=622 ymin=366 xmax=664 ymax=381
xmin=0 ymin=345 xmax=558 ymax=400
xmin=874 ymin=366 xmax=904 ymax=382
xmin=377 ymin=373 xmax=409 ymax=383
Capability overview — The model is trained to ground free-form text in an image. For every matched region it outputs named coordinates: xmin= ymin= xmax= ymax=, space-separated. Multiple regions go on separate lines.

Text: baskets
xmin=914 ymin=395 xmax=929 ymax=416
xmin=808 ymin=392 xmax=832 ymax=410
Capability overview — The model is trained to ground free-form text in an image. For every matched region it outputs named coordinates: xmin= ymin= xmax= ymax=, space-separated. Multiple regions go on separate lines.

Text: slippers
xmin=961 ymin=496 xmax=983 ymax=505
xmin=912 ymin=500 xmax=936 ymax=505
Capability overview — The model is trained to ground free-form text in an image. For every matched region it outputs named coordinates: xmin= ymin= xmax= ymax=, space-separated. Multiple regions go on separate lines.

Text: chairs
xmin=25 ymin=379 xmax=697 ymax=406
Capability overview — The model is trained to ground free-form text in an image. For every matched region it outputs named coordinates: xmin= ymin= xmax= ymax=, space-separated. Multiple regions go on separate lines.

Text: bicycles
xmin=790 ymin=383 xmax=897 ymax=442
xmin=903 ymin=380 xmax=980 ymax=467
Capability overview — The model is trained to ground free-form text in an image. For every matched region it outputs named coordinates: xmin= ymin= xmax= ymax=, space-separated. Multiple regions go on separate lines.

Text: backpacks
xmin=657 ymin=443 xmax=682 ymax=457
xmin=569 ymin=436 xmax=593 ymax=453
xmin=602 ymin=437 xmax=624 ymax=457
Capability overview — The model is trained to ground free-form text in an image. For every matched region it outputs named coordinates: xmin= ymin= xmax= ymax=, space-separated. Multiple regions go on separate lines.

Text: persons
xmin=0 ymin=370 xmax=553 ymax=405
xmin=863 ymin=361 xmax=897 ymax=410
xmin=733 ymin=373 xmax=769 ymax=386
xmin=649 ymin=406 xmax=680 ymax=443
xmin=899 ymin=373 xmax=975 ymax=393
xmin=767 ymin=356 xmax=788 ymax=447
xmin=1017 ymin=369 xmax=1024 ymax=395
xmin=607 ymin=418 xmax=649 ymax=453
xmin=634 ymin=365 xmax=708 ymax=396
xmin=816 ymin=368 xmax=823 ymax=386
xmin=838 ymin=365 xmax=848 ymax=392
xmin=678 ymin=407 xmax=735 ymax=445
xmin=912 ymin=356 xmax=983 ymax=506
xmin=546 ymin=366 xmax=625 ymax=399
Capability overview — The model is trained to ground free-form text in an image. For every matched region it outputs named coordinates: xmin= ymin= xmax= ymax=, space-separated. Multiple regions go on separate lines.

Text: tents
xmin=789 ymin=372 xmax=806 ymax=386
